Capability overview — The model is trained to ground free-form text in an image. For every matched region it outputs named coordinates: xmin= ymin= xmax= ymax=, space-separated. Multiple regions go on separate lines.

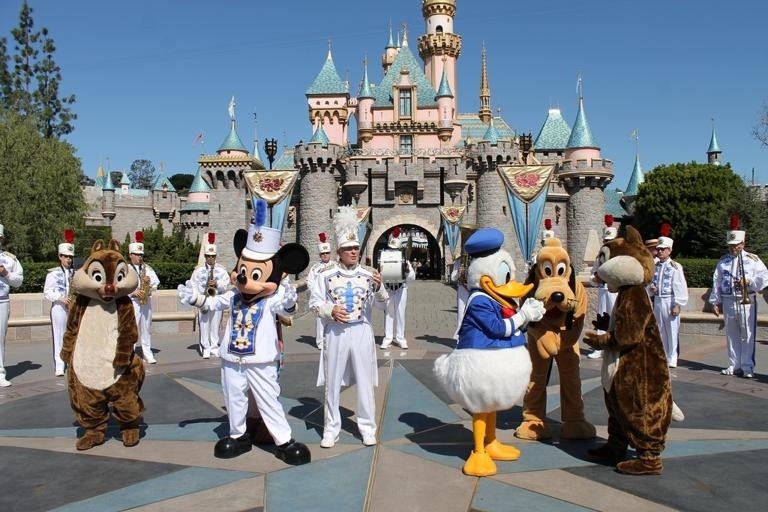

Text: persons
xmin=125 ymin=253 xmax=162 ymax=364
xmin=42 ymin=254 xmax=78 ymax=377
xmin=586 ymin=238 xmax=617 ymax=359
xmin=188 ymin=255 xmax=230 ymax=359
xmin=451 ymin=254 xmax=473 ymax=332
xmin=710 ymin=242 xmax=768 ymax=379
xmin=645 ymin=249 xmax=688 ymax=368
xmin=526 ymin=240 xmax=545 ymax=270
xmin=645 ymin=238 xmax=662 ymax=307
xmin=308 ymin=245 xmax=391 ymax=449
xmin=0 ymin=224 xmax=25 ymax=388
xmin=403 ymin=235 xmax=439 ymax=279
xmin=305 ymin=251 xmax=341 ymax=350
xmin=376 ymin=248 xmax=415 ymax=350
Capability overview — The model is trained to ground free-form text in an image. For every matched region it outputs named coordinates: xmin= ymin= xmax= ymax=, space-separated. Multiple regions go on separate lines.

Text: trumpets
xmin=737 ymin=246 xmax=751 ymax=304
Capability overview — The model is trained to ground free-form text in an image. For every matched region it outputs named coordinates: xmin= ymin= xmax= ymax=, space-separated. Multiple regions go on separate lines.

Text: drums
xmin=377 ymin=248 xmax=407 ymax=284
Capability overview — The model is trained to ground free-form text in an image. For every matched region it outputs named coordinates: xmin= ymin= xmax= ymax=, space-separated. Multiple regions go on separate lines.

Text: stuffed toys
xmin=59 ymin=237 xmax=149 ymax=449
xmin=178 ymin=222 xmax=313 ymax=465
xmin=432 ymin=227 xmax=548 ymax=477
xmin=582 ymin=224 xmax=673 ymax=475
xmin=512 ymin=236 xmax=597 ymax=441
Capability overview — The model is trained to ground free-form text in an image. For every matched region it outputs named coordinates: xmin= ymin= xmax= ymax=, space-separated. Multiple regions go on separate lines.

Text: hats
xmin=58 ymin=242 xmax=74 ymax=257
xmin=128 ymin=243 xmax=144 ymax=254
xmin=388 ymin=238 xmax=400 ymax=249
xmin=602 ymin=228 xmax=617 ymax=240
xmin=204 ymin=244 xmax=217 ymax=256
xmin=656 ymin=236 xmax=674 ymax=251
xmin=317 ymin=243 xmax=331 ymax=255
xmin=726 ymin=230 xmax=746 ymax=245
xmin=645 ymin=239 xmax=659 ymax=248
xmin=334 ymin=231 xmax=360 ymax=250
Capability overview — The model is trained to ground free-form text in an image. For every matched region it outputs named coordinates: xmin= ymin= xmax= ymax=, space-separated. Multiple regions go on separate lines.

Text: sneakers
xmin=721 ymin=368 xmax=741 ymax=375
xmin=55 ymin=371 xmax=65 ymax=377
xmin=0 ymin=379 xmax=11 ymax=387
xmin=587 ymin=350 xmax=601 ymax=358
xmin=320 ymin=436 xmax=339 ymax=447
xmin=203 ymin=352 xmax=211 ymax=359
xmin=142 ymin=358 xmax=157 ymax=364
xmin=363 ymin=435 xmax=377 ymax=446
xmin=742 ymin=371 xmax=755 ymax=378
xmin=380 ymin=340 xmax=409 ymax=349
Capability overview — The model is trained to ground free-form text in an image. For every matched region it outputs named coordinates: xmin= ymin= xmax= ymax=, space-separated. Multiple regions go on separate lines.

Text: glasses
xmin=657 ymin=248 xmax=667 ymax=251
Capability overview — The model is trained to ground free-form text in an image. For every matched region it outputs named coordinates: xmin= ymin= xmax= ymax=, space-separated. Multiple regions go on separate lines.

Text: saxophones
xmin=201 ymin=259 xmax=218 ymax=313
xmin=66 ymin=264 xmax=75 ymax=311
xmin=138 ymin=258 xmax=152 ymax=305
xmin=458 ymin=223 xmax=480 ymax=286
